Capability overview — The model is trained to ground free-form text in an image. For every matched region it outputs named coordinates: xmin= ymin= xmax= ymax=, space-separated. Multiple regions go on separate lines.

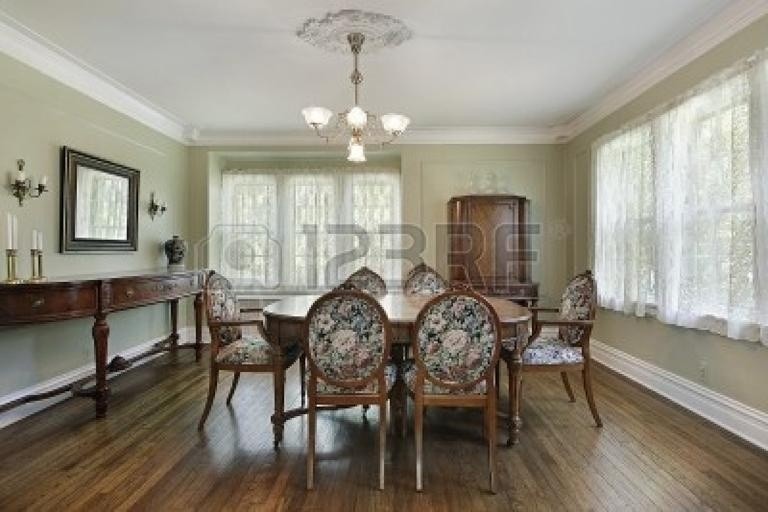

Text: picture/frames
xmin=58 ymin=144 xmax=141 ymax=255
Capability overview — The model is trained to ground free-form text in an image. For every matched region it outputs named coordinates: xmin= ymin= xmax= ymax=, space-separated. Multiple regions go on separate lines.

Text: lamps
xmin=302 ymin=32 xmax=411 ymax=163
xmin=9 ymin=158 xmax=49 ymax=206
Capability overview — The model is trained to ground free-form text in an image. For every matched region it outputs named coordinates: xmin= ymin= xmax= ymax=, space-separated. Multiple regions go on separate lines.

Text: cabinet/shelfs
xmin=0 ymin=270 xmax=211 ymax=420
xmin=445 ymin=193 xmax=531 ymax=306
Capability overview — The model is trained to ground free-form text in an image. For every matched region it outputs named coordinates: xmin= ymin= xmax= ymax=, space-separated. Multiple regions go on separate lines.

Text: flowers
xmin=163 ymin=234 xmax=188 ymax=265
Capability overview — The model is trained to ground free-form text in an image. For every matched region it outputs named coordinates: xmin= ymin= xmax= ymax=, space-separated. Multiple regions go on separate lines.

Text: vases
xmin=167 ymin=263 xmax=185 ymax=274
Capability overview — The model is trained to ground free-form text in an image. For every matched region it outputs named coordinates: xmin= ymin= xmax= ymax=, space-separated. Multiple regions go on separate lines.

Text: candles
xmin=148 ymin=191 xmax=167 ymax=222
xmin=30 ymin=228 xmax=43 ymax=250
xmin=5 ymin=212 xmax=18 ymax=248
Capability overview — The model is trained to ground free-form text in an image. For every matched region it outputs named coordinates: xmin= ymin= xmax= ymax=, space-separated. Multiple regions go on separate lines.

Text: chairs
xmin=402 ymin=263 xmax=450 ymax=361
xmin=304 ymin=287 xmax=398 ymax=490
xmin=332 ymin=266 xmax=397 ymax=419
xmin=494 ymin=270 xmax=604 ymax=446
xmin=401 ymin=288 xmax=502 ymax=495
xmin=197 ymin=271 xmax=308 ymax=447
